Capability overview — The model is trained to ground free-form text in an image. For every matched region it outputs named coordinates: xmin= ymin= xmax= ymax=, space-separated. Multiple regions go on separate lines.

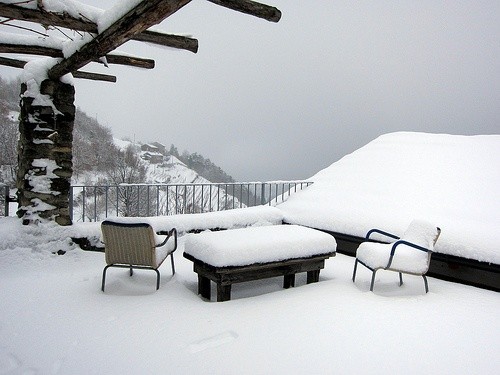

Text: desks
xmin=183 ymin=249 xmax=337 ymax=302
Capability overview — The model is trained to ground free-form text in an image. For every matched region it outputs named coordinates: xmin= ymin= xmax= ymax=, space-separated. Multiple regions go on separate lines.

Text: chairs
xmin=350 ymin=219 xmax=441 ymax=294
xmin=100 ymin=219 xmax=177 ymax=294
